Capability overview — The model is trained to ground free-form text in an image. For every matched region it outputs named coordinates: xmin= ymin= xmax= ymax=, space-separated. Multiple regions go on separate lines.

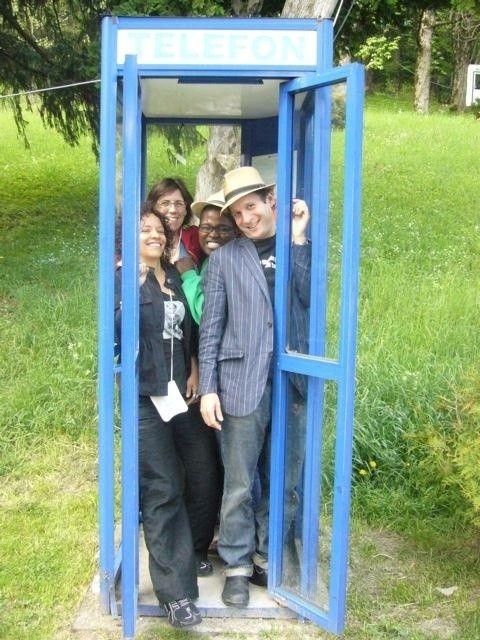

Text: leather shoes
xmin=246 ymin=564 xmax=267 ymax=586
xmin=222 ymin=574 xmax=249 ymax=608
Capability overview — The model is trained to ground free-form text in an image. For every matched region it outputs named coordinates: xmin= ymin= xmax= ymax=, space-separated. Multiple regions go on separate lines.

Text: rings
xmin=192 ymin=394 xmax=196 ymax=398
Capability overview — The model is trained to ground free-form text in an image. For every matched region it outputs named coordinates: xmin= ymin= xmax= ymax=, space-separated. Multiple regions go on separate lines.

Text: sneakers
xmin=195 ymin=556 xmax=213 ymax=576
xmin=162 ymin=597 xmax=201 ymax=628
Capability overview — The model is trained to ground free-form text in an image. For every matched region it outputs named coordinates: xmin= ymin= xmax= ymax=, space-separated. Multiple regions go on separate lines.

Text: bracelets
xmin=293 ymin=239 xmax=309 ymax=245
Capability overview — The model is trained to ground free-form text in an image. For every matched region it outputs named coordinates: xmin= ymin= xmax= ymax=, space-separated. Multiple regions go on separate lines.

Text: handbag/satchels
xmin=149 ymin=379 xmax=188 ymax=423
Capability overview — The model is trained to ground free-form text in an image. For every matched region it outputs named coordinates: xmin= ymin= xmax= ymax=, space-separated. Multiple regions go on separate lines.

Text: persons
xmin=147 ymin=178 xmax=208 ymax=275
xmin=197 ymin=166 xmax=312 ymax=610
xmin=114 ymin=205 xmax=221 ymax=626
xmin=173 ymin=188 xmax=239 ymax=326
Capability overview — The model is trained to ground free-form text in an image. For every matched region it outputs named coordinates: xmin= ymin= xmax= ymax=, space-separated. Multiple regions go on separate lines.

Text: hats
xmin=190 ymin=189 xmax=225 ymax=219
xmin=219 ymin=166 xmax=276 ymax=217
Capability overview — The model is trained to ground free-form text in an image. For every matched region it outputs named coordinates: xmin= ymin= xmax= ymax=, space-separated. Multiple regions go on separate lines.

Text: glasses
xmin=198 ymin=224 xmax=235 ymax=234
xmin=157 ymin=200 xmax=187 ymax=209
